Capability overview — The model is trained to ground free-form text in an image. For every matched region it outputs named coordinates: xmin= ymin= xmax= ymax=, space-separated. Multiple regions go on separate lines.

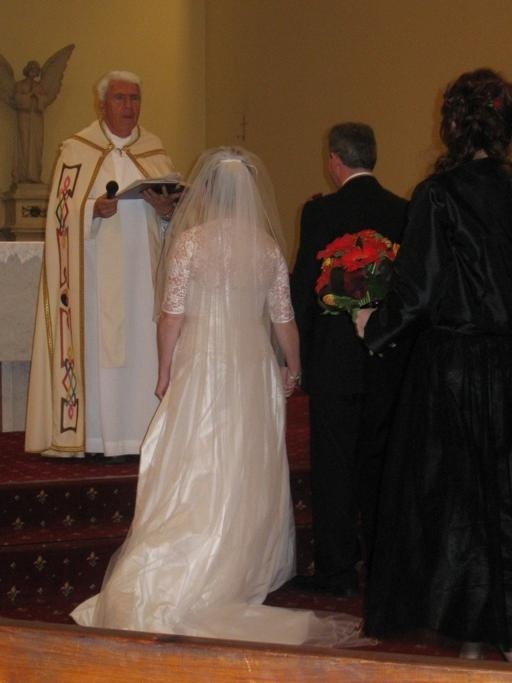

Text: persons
xmin=287 ymin=121 xmax=416 ymax=595
xmin=23 ymin=68 xmax=184 ymax=463
xmin=151 ymin=143 xmax=304 ymax=607
xmin=346 ymin=67 xmax=510 ymax=667
xmin=14 ymin=59 xmax=51 ymax=182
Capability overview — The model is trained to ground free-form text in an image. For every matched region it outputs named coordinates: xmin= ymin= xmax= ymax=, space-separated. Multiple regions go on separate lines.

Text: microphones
xmin=106 ymin=180 xmax=119 ymax=199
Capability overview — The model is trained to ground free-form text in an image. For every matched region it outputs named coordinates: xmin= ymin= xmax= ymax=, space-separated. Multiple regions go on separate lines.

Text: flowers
xmin=315 ymin=230 xmax=402 ymax=326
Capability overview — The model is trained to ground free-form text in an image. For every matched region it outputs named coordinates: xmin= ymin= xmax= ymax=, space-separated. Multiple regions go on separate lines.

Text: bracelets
xmin=286 ymin=367 xmax=303 ymax=381
xmin=157 ymin=206 xmax=177 ymax=218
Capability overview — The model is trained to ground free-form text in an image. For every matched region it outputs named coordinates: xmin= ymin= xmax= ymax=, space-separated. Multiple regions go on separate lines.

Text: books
xmin=114 ymin=176 xmax=189 ymax=200
